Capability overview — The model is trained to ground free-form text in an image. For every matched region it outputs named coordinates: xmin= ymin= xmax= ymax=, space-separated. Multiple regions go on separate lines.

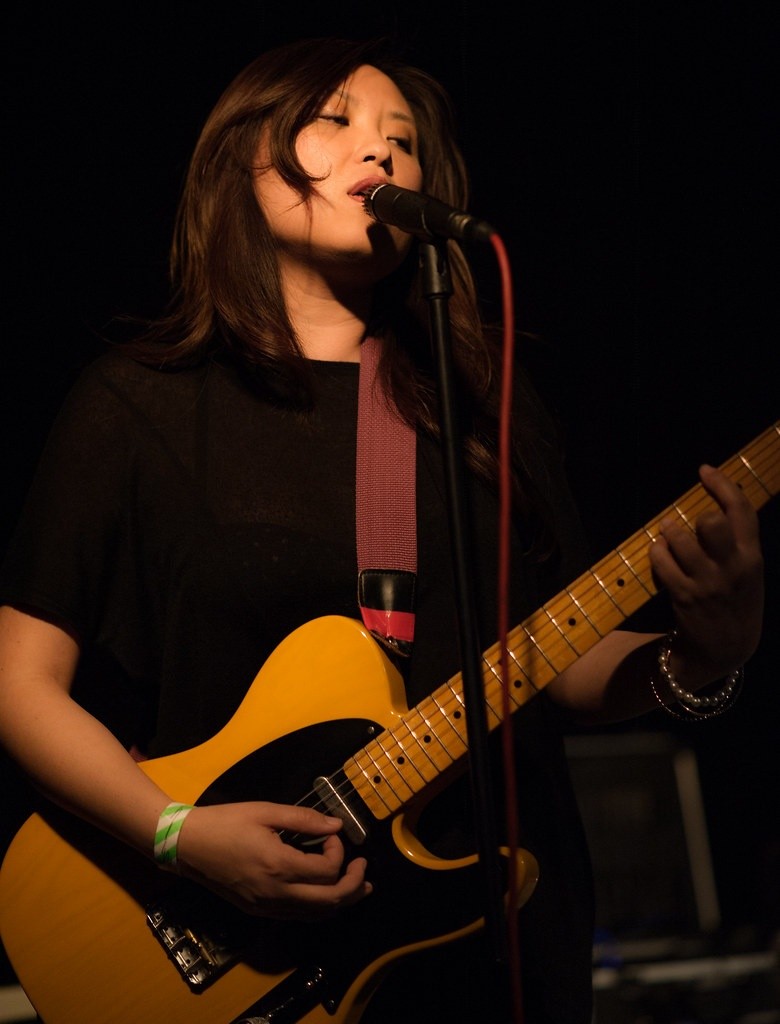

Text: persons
xmin=0 ymin=40 xmax=766 ymax=1023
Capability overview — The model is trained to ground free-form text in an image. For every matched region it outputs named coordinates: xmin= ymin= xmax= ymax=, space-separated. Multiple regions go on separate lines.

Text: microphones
xmin=361 ymin=181 xmax=478 ymax=243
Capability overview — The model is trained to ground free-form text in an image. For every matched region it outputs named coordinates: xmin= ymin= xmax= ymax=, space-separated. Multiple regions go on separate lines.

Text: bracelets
xmin=154 ymin=803 xmax=197 ymax=866
xmin=649 ymin=628 xmax=744 ymax=722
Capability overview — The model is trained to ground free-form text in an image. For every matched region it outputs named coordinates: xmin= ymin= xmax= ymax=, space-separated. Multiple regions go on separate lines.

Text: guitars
xmin=0 ymin=415 xmax=780 ymax=1024
